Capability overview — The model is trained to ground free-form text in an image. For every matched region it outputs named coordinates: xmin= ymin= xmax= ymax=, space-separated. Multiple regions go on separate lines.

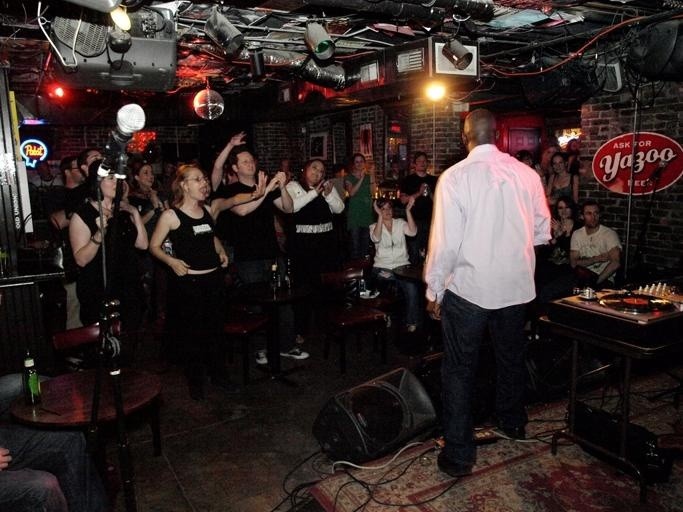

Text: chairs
xmin=214 ymin=266 xmax=274 ymax=380
xmin=45 ymin=315 xmax=164 ymax=463
xmin=320 ymin=263 xmax=386 ymax=369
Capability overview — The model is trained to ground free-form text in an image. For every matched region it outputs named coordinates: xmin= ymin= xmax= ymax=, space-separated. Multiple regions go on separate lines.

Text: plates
xmin=576 ymin=295 xmax=598 ymax=301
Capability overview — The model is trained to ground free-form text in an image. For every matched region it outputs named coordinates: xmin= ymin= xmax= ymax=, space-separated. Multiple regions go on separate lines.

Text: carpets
xmin=307 ymin=362 xmax=683 ymax=512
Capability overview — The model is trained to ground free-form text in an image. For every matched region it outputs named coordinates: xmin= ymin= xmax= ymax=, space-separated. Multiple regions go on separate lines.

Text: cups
xmin=585 ymin=287 xmax=592 ymax=298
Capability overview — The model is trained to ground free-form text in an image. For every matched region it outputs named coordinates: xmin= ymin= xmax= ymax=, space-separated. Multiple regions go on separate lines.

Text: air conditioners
xmin=276 ymin=38 xmax=482 ymax=112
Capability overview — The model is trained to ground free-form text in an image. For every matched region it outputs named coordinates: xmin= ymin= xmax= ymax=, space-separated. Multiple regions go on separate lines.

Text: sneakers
xmin=255 ymin=351 xmax=268 ymax=364
xmin=280 ymin=347 xmax=310 ymax=359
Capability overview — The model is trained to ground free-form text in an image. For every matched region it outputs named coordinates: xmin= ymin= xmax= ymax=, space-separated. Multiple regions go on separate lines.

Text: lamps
xmin=197 ymin=3 xmax=474 ymax=77
xmin=68 ymin=0 xmax=134 ymax=33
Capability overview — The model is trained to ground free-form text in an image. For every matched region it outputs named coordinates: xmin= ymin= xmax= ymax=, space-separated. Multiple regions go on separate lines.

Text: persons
xmin=513 ymin=138 xmax=622 ymax=305
xmin=422 ymin=108 xmax=552 ymax=477
xmin=0 ymin=423 xmax=111 ymax=512
xmin=50 ymin=130 xmax=437 ymax=365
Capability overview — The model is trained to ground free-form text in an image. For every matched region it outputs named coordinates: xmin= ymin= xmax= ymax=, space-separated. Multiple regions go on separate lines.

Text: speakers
xmin=417 ymin=353 xmax=501 ymax=421
xmin=523 ymin=338 xmax=610 ymax=402
xmin=312 ymin=368 xmax=438 ymax=468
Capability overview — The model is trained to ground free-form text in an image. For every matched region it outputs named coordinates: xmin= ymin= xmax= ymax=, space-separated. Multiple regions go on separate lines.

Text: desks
xmin=0 ymin=366 xmax=166 ymax=512
xmin=537 ymin=279 xmax=682 ymax=503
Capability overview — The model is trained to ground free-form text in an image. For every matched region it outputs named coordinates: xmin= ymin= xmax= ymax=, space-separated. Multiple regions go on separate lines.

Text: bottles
xmin=638 ymin=281 xmax=679 ymax=302
xmin=21 ymin=348 xmax=42 ymax=407
xmin=268 ymin=256 xmax=295 ymax=301
xmin=372 ymin=184 xmax=397 ymax=200
xmin=0 ymin=244 xmax=9 ymax=277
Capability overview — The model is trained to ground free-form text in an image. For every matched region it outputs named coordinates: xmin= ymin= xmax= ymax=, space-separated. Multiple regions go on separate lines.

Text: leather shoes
xmin=437 ymin=452 xmax=472 ymax=477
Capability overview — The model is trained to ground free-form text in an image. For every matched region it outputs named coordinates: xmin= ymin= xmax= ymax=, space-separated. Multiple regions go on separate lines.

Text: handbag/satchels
xmin=16 ymin=211 xmax=65 ymax=276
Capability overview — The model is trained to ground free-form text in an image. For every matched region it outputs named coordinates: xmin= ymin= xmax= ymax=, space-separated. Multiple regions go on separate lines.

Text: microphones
xmin=645 ymin=161 xmax=666 ymax=182
xmin=96 ymin=103 xmax=146 ymax=188
